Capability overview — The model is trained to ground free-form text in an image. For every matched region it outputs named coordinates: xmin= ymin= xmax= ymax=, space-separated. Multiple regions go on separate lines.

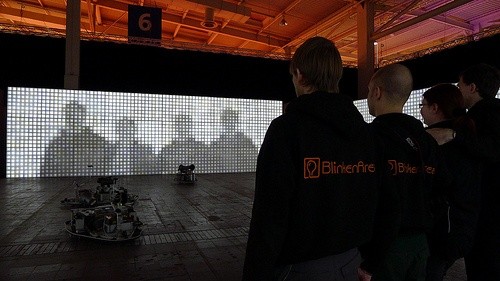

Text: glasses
xmin=419 ymin=104 xmax=429 ymax=108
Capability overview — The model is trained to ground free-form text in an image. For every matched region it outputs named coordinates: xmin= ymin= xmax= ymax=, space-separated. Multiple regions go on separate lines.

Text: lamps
xmin=280 ymin=12 xmax=288 ymax=27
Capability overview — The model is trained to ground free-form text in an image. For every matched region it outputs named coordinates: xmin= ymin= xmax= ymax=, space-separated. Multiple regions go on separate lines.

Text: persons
xmin=242 ymin=36 xmax=374 ymax=280
xmin=457 ymin=62 xmax=500 ymax=281
xmin=420 ymin=83 xmax=478 ymax=281
xmin=362 ymin=63 xmax=440 ymax=281
